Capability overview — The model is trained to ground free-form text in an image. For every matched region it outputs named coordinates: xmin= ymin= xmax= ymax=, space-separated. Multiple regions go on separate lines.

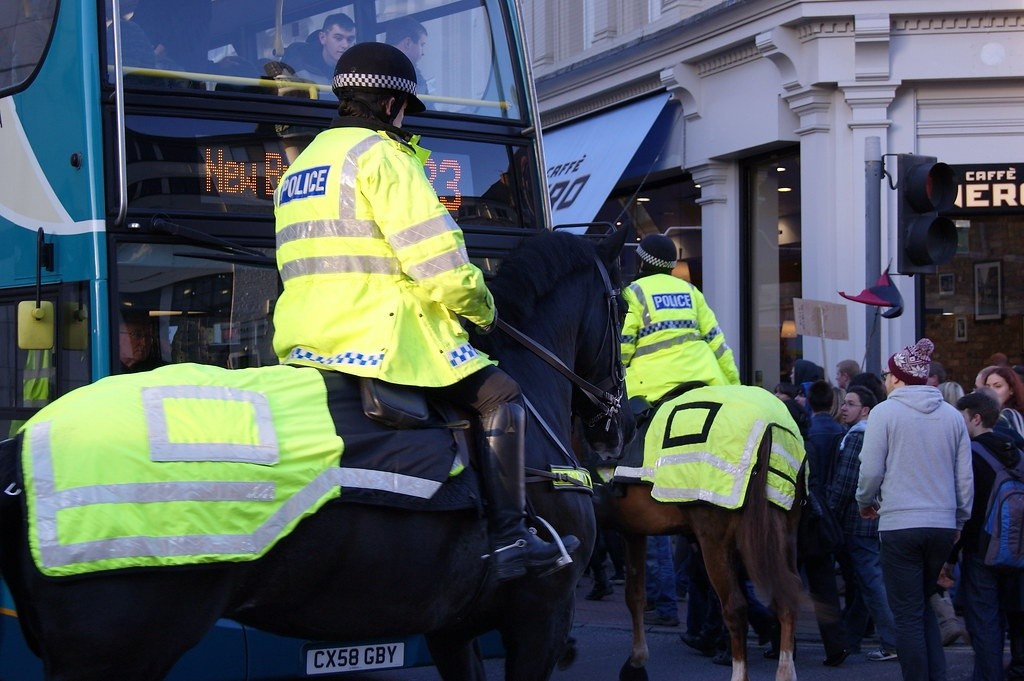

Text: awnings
xmin=543 ymin=93 xmax=675 ymax=239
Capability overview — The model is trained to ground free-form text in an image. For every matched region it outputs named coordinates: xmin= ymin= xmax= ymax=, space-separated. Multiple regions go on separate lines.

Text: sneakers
xmin=644 ymin=611 xmax=679 ymax=625
xmin=867 ymin=646 xmax=898 ymax=661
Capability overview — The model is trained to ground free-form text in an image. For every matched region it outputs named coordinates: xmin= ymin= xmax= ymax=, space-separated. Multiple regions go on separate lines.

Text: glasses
xmin=881 ymin=371 xmax=893 ymax=381
xmin=120 ymin=330 xmax=154 ymax=345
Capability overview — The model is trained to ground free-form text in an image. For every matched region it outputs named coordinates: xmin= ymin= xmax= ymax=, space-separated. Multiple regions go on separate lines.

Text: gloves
xmin=476 ymin=305 xmax=499 ymax=335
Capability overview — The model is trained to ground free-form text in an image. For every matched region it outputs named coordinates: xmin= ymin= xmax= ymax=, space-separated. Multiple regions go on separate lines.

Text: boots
xmin=479 ymin=403 xmax=580 ymax=581
xmin=585 ymin=566 xmax=614 ymax=599
xmin=929 ymin=591 xmax=963 ymax=646
xmin=609 ymin=564 xmax=625 ymax=583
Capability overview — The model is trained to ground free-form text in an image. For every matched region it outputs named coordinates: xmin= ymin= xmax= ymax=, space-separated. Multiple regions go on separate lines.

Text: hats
xmin=889 ymin=338 xmax=934 ymax=384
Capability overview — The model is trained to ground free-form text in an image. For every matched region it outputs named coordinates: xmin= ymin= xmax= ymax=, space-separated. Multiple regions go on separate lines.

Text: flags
xmin=838 ymin=267 xmax=904 ymax=319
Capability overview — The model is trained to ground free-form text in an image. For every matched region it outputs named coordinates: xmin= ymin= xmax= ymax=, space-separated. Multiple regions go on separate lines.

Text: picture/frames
xmin=936 ymin=272 xmax=956 ymax=297
xmin=954 ymin=316 xmax=968 ymax=342
xmin=972 ymin=259 xmax=1005 ymax=324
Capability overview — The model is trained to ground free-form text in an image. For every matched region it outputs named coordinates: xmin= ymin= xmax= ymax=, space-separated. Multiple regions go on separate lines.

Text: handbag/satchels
xmin=798 ymin=497 xmax=826 ymax=564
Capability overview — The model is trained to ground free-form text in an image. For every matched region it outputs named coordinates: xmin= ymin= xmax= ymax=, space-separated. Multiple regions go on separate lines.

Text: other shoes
xmin=593 ymin=472 xmax=607 ymax=506
xmin=764 ymin=650 xmax=776 ymax=659
xmin=824 ymin=649 xmax=850 ymax=666
xmin=680 ymin=634 xmax=716 ymax=656
xmin=711 ymin=651 xmax=732 ymax=665
xmin=759 ymin=616 xmax=771 ymax=646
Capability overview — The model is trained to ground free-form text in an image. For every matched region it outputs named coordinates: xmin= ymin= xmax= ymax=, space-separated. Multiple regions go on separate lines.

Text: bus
xmin=0 ymin=0 xmax=578 ymax=681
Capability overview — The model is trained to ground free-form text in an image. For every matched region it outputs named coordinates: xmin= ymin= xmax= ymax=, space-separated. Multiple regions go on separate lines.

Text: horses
xmin=0 ymin=220 xmax=635 ymax=681
xmin=583 ymin=389 xmax=818 ymax=681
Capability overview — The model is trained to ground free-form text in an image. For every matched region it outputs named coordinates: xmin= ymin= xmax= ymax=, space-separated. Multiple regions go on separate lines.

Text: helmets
xmin=332 ymin=42 xmax=426 ymax=117
xmin=636 ymin=234 xmax=677 ymax=269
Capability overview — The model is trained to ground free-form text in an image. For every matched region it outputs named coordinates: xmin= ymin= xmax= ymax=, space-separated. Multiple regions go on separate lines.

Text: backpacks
xmin=971 ymin=440 xmax=1024 ymax=571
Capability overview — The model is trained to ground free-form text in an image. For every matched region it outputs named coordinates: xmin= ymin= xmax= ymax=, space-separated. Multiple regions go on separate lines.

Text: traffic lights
xmin=894 ymin=152 xmax=962 ymax=275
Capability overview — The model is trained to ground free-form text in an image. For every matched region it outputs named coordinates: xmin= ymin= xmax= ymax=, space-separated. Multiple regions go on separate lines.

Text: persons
xmin=106 ymin=0 xmax=213 ymax=70
xmin=587 ymin=535 xmax=736 ymax=664
xmin=273 ymin=39 xmax=580 ymax=581
xmin=581 ymin=235 xmax=740 ymax=560
xmin=855 ymin=339 xmax=974 ymax=681
xmin=386 ymin=17 xmax=435 ymax=111
xmin=931 ymin=354 xmax=1024 ymax=681
xmin=744 ymin=360 xmax=898 ymax=665
xmin=281 ymin=13 xmax=356 ymax=84
xmin=944 ymin=393 xmax=1024 ymax=681
xmin=117 ymin=306 xmax=167 ymax=374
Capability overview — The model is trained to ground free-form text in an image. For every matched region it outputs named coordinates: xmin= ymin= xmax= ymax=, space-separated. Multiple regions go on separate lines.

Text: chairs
xmin=213 ymin=53 xmax=260 ymax=78
